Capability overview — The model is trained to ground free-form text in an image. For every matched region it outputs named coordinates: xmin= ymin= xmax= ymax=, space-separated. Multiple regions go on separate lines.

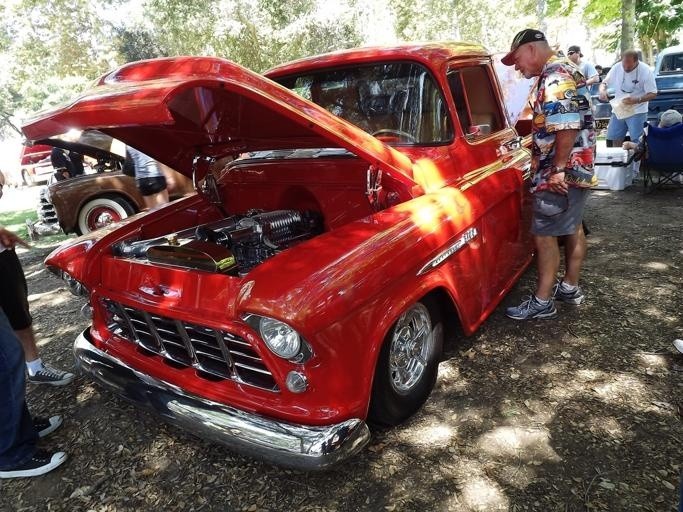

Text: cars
xmin=588 ymin=68 xmax=613 ymax=120
xmin=647 ymin=46 xmax=683 ymax=120
xmin=20 ymin=144 xmax=56 ymax=188
xmin=25 ymin=139 xmax=235 ymax=241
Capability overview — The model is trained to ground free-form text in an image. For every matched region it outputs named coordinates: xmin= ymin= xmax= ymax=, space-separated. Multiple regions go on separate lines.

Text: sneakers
xmin=28 ymin=363 xmax=76 ymax=385
xmin=553 ymin=277 xmax=585 ymax=306
xmin=31 ymin=414 xmax=63 ymax=437
xmin=506 ymin=294 xmax=558 ymax=321
xmin=0 ymin=450 xmax=69 ymax=478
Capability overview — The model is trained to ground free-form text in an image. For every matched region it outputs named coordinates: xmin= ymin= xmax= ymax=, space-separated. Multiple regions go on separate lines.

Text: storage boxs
xmin=588 ymin=147 xmax=643 ymax=191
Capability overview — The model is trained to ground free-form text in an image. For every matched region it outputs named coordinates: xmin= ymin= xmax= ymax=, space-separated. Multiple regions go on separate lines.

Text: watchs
xmin=638 ymin=96 xmax=642 ymax=103
xmin=551 ymin=166 xmax=564 ymax=174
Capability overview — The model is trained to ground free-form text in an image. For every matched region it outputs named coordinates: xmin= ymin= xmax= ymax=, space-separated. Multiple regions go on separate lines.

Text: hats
xmin=501 ymin=29 xmax=545 ymax=66
xmin=658 ymin=109 xmax=682 ymax=128
xmin=569 ymin=46 xmax=583 ymax=57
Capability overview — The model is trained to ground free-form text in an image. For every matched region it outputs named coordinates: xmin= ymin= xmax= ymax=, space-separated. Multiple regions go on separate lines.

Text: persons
xmin=567 ymin=45 xmax=600 ymax=91
xmin=501 ymin=28 xmax=598 ymax=321
xmin=0 ymin=170 xmax=76 ymax=386
xmin=125 ymin=144 xmax=176 ymax=210
xmin=622 ymin=109 xmax=682 ymax=160
xmin=1 ymin=224 xmax=68 ymax=479
xmin=595 ymin=65 xmax=607 ymax=76
xmin=598 ymin=49 xmax=658 ymax=148
xmin=51 ymin=146 xmax=121 ymax=181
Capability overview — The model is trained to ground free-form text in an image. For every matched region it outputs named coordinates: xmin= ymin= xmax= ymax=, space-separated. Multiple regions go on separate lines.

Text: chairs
xmin=641 ymin=123 xmax=682 ymax=196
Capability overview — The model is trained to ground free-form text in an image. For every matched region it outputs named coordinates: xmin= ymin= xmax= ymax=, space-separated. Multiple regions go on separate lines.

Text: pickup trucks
xmin=19 ymin=38 xmax=533 ymax=471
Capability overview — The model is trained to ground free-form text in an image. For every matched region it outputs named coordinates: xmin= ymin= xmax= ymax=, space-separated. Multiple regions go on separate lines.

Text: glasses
xmin=621 ymin=81 xmax=636 ymax=93
